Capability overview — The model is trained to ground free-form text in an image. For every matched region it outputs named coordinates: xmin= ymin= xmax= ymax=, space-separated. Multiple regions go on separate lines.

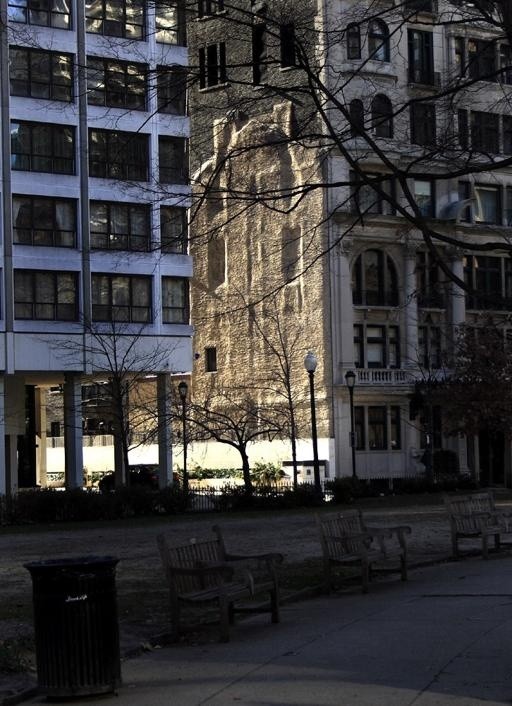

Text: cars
xmin=97 ymin=462 xmax=181 ymax=492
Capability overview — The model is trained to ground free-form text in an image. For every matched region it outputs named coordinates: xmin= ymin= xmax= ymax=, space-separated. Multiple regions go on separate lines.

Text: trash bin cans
xmin=23 ymin=555 xmax=122 ymax=703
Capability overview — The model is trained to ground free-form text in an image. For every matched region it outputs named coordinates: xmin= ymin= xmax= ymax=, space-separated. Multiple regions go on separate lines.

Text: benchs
xmin=314 ymin=505 xmax=412 ymax=595
xmin=442 ymin=490 xmax=511 ymax=559
xmin=154 ymin=522 xmax=283 ymax=643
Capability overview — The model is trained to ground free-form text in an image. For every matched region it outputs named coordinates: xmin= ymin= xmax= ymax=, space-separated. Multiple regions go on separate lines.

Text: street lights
xmin=343 ymin=369 xmax=357 ymax=479
xmin=303 ymin=350 xmax=321 ymax=486
xmin=177 ymin=380 xmax=190 ymax=492
